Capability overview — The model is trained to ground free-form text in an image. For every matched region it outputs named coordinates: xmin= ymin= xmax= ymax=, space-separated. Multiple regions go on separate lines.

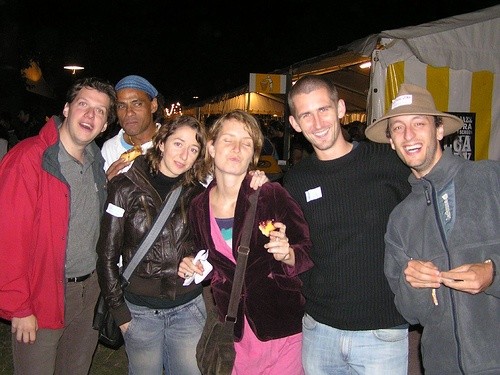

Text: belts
xmin=68 ymin=270 xmax=95 ymax=282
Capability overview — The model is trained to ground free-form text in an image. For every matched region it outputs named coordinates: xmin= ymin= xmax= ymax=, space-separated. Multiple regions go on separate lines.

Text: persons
xmin=176 ymin=109 xmax=312 ymax=375
xmin=96 ymin=114 xmax=269 ymax=375
xmin=0 ymin=75 xmax=118 ymax=375
xmin=100 ymin=75 xmax=162 ymax=182
xmin=281 ymin=73 xmax=412 ymax=375
xmin=364 ymin=83 xmax=500 ymax=375
xmin=205 ymin=112 xmax=371 ymax=172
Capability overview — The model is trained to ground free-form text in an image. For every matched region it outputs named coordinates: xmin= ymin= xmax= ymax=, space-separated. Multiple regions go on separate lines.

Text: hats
xmin=115 ymin=75 xmax=158 ymax=98
xmin=365 ymin=83 xmax=464 ymax=143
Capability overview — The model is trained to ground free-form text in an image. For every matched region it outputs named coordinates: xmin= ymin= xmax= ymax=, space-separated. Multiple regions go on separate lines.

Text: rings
xmin=275 ymin=236 xmax=281 ymax=241
xmin=184 ymin=271 xmax=190 ymax=278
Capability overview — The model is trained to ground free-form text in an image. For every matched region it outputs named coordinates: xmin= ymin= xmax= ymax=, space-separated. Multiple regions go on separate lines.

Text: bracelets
xmin=282 ymin=252 xmax=291 ymax=261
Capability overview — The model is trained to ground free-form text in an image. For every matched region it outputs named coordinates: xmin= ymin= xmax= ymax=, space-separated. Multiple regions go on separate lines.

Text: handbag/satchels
xmin=92 ymin=291 xmax=125 ymax=350
xmin=196 ymin=308 xmax=236 ymax=375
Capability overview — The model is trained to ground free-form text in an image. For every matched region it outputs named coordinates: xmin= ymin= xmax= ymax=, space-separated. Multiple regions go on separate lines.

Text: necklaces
xmin=128 ymin=135 xmax=136 ymax=145
